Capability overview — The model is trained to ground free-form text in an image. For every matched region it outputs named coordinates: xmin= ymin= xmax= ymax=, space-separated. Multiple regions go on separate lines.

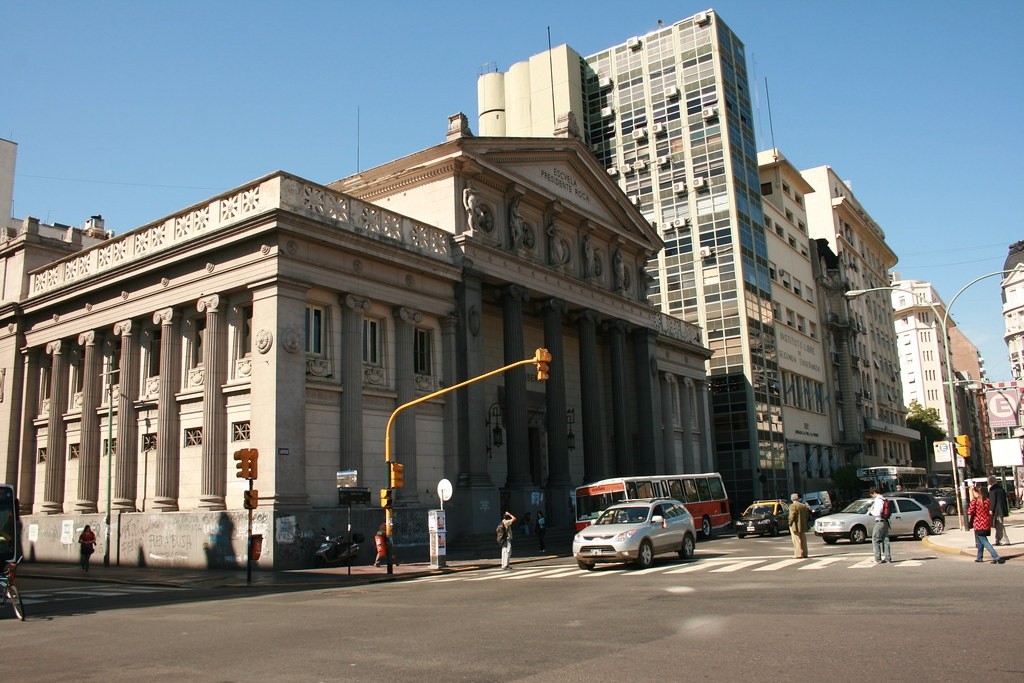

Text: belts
xmin=876 ymin=520 xmax=887 ymax=522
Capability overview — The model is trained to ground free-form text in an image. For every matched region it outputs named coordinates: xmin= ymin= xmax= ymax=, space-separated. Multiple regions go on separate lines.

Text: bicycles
xmin=0 ymin=555 xmax=27 ymax=622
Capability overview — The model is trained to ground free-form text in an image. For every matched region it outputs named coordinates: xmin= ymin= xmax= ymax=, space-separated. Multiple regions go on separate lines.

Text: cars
xmin=735 ymin=499 xmax=790 ymax=540
xmin=814 ymin=496 xmax=933 ymax=545
xmin=938 ymin=486 xmax=969 ymax=516
xmin=882 ymin=492 xmax=947 ymax=535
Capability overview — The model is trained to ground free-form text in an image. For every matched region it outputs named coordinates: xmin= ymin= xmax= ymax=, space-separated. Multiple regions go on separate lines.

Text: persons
xmin=789 ymin=494 xmax=808 ymax=558
xmin=969 ymin=487 xmax=1001 ymax=565
xmin=534 ymin=511 xmax=547 ymax=552
xmin=78 ymin=525 xmax=96 ymax=571
xmin=625 ymin=513 xmax=633 ymax=521
xmin=988 ymin=476 xmax=1010 ymax=546
xmin=501 ymin=511 xmax=517 ymax=569
xmin=0 ymin=508 xmax=13 ymax=553
xmin=373 ymin=522 xmax=400 ymax=567
xmin=867 ymin=487 xmax=891 ymax=564
xmin=518 ymin=512 xmax=534 ymax=535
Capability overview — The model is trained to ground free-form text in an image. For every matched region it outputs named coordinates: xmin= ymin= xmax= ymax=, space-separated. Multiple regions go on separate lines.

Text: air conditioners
xmin=661 ymin=222 xmax=671 ymax=231
xmin=673 ymin=182 xmax=685 ymax=193
xmin=674 ymin=217 xmax=686 ymax=228
xmin=665 ymin=85 xmax=677 ymax=97
xmin=651 ymin=122 xmax=663 ymax=134
xmin=699 ymin=246 xmax=710 ymax=257
xmin=628 ymin=195 xmax=638 ymax=205
xmin=620 ymin=164 xmax=631 ymax=173
xmin=702 ymin=107 xmax=714 ymax=118
xmin=599 ymin=77 xmax=611 ymax=87
xmin=600 ymin=106 xmax=613 ymax=118
xmin=656 ymin=156 xmax=667 ymax=164
xmin=634 ymin=160 xmax=645 ymax=170
xmin=692 ymin=176 xmax=704 ymax=188
xmin=626 ymin=36 xmax=639 ymax=48
xmin=607 ymin=168 xmax=617 ymax=175
xmin=632 ymin=128 xmax=645 ymax=139
xmin=694 ymin=11 xmax=707 ymax=23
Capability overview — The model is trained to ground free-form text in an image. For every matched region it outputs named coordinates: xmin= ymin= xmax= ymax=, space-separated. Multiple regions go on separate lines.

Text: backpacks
xmin=496 ymin=521 xmax=509 ymax=544
xmin=880 ymin=498 xmax=889 ymax=518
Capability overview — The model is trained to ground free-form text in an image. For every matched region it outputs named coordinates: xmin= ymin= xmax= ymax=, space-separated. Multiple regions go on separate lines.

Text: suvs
xmin=572 ymin=496 xmax=698 ymax=570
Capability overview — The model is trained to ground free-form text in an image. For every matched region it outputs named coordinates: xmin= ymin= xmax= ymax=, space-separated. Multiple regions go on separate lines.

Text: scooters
xmin=315 ymin=527 xmax=365 ymax=568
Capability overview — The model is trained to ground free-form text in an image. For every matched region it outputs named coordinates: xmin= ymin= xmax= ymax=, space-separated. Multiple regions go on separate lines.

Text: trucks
xmin=804 ymin=491 xmax=833 ymax=518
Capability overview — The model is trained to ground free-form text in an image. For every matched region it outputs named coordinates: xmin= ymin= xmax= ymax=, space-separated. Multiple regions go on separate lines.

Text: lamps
xmin=566 ymin=408 xmax=575 ymax=452
xmin=485 ymin=402 xmax=504 ymax=460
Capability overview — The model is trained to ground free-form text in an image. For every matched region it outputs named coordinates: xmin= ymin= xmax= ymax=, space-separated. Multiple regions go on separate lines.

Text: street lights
xmin=844 ymin=268 xmax=1024 ymax=533
xmin=103 ymin=383 xmax=113 ymax=569
xmin=942 ymin=379 xmax=1024 ymax=509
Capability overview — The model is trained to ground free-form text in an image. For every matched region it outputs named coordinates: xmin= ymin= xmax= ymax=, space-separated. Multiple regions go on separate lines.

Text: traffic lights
xmin=243 ymin=489 xmax=259 ymax=509
xmin=380 ymin=489 xmax=392 ymax=509
xmin=536 ymin=348 xmax=553 ymax=382
xmin=390 ymin=462 xmax=405 ymax=488
xmin=234 ymin=447 xmax=250 ymax=479
xmin=247 ymin=448 xmax=258 ymax=480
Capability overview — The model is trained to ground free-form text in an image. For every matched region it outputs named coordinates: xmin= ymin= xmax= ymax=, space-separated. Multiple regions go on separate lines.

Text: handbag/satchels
xmin=969 ymin=498 xmax=977 ymax=529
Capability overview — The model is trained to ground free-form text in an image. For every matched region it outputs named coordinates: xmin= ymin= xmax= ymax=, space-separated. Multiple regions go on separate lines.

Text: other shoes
xmin=992 ymin=558 xmax=1001 ymax=563
xmin=975 ymin=558 xmax=983 ymax=563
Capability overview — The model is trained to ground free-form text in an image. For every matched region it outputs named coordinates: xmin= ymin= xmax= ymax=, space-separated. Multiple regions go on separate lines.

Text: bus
xmin=963 ymin=476 xmax=1017 ymax=509
xmin=574 ymin=471 xmax=732 ymax=540
xmin=855 ymin=466 xmax=928 ymax=494
xmin=0 ymin=484 xmax=20 ymax=563
